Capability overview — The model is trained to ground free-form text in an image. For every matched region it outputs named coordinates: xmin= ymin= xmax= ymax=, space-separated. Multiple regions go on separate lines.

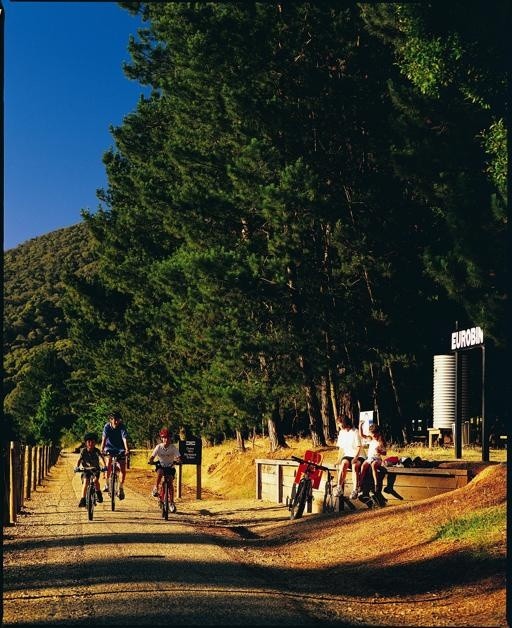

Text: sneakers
xmin=95 ymin=490 xmax=103 ymax=502
xmin=78 ymin=498 xmax=86 ymax=507
xmin=119 ymin=488 xmax=125 ymax=500
xmin=153 ymin=488 xmax=160 ymax=496
xmin=103 ymin=484 xmax=109 ymax=492
xmin=169 ymin=503 xmax=176 ymax=512
xmin=333 ymin=485 xmax=382 ymax=498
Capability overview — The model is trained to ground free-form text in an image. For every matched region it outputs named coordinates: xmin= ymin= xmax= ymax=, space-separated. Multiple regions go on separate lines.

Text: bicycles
xmin=289 ymin=455 xmax=329 ymax=520
xmin=101 ymin=452 xmax=126 ymax=512
xmin=147 ymin=461 xmax=183 ymax=520
xmin=74 ymin=466 xmax=108 ymax=520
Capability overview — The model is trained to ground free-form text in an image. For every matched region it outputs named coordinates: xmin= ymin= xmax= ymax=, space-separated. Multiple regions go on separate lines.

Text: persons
xmin=332 ymin=414 xmax=366 ymax=499
xmin=356 ymin=420 xmax=387 ymax=494
xmin=75 ymin=432 xmax=106 ymax=507
xmin=100 ymin=412 xmax=129 ymax=501
xmin=149 ymin=429 xmax=182 ymax=513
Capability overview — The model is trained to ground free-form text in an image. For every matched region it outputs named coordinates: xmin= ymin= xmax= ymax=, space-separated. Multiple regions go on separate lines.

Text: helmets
xmin=160 ymin=428 xmax=171 ymax=437
xmin=107 ymin=413 xmax=122 ymax=420
xmin=84 ymin=434 xmax=97 ymax=442
